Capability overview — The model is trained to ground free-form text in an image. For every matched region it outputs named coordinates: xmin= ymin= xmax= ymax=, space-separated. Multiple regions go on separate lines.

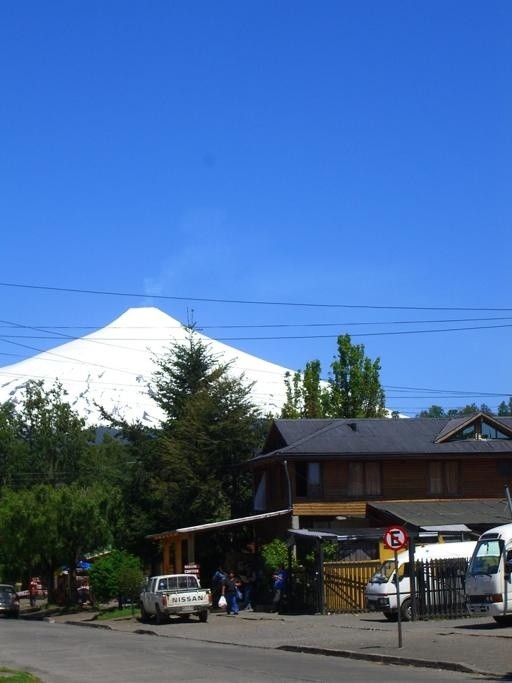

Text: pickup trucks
xmin=139 ymin=572 xmax=212 ymax=622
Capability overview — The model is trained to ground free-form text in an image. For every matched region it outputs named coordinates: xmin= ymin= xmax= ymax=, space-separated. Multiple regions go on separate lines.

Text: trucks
xmin=461 ymin=522 xmax=511 ymax=621
xmin=365 ymin=539 xmax=478 ymax=622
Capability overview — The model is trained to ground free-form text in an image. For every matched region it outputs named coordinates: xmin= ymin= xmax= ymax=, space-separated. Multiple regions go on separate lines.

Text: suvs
xmin=0 ymin=584 xmax=20 ymax=618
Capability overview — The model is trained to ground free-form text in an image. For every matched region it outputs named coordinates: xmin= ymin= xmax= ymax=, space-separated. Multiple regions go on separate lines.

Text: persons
xmin=268 ymin=563 xmax=288 ymax=614
xmin=211 ymin=565 xmax=259 ymax=616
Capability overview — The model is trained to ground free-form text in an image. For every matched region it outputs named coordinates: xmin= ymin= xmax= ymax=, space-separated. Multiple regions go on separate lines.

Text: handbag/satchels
xmin=236 ymin=589 xmax=243 ymax=600
